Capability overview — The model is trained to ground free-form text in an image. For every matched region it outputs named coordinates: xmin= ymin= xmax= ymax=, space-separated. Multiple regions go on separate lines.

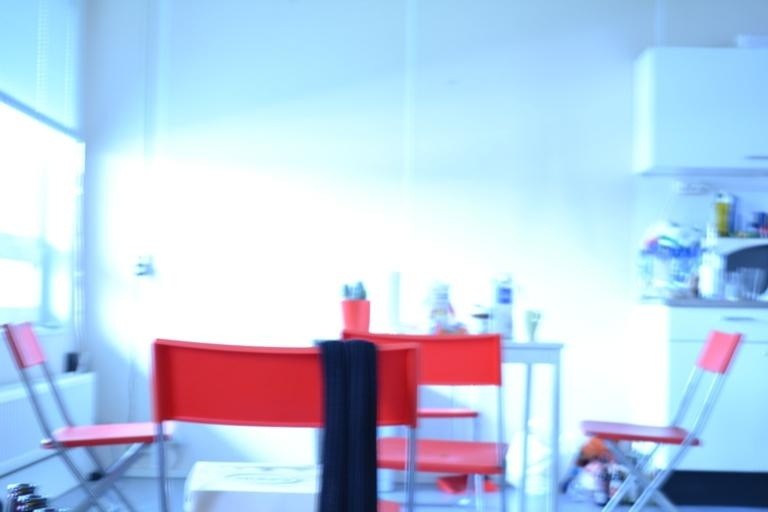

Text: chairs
xmin=2 ymin=319 xmax=174 ymax=511
xmin=578 ymin=326 xmax=747 ymax=510
xmin=149 ymin=319 xmax=564 ymax=512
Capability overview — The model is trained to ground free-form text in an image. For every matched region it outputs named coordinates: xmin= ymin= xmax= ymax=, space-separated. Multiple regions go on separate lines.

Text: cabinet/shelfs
xmin=632 ymin=47 xmax=768 ymax=174
xmin=665 ymin=306 xmax=767 ymax=474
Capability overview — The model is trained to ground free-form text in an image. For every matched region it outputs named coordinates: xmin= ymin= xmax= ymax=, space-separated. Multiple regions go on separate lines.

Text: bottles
xmin=494 ymin=271 xmax=516 ymax=341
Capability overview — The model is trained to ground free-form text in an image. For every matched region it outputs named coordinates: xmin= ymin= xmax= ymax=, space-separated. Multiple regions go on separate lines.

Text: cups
xmin=735 ymin=266 xmax=760 ymax=301
xmin=522 ymin=307 xmax=542 ymax=344
xmin=340 ymin=299 xmax=371 ymax=336
